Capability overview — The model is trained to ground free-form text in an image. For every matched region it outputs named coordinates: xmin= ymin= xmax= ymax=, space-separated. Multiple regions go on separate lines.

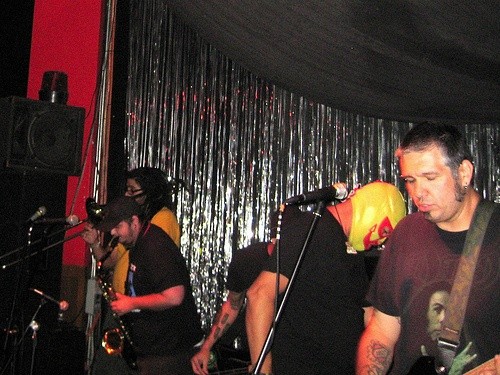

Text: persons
xmin=190 ymin=204 xmax=302 ymax=375
xmin=81 ymin=167 xmax=180 ymax=375
xmin=355 ymin=122 xmax=500 ymax=375
xmin=246 ymin=180 xmax=407 ymax=375
xmin=401 ymin=279 xmax=450 ymax=358
xmin=94 ymin=196 xmax=206 ymax=375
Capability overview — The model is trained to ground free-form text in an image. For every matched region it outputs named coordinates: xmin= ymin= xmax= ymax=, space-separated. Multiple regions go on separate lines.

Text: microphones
xmin=0 ymin=96 xmax=86 ymax=177
xmin=25 ymin=206 xmax=47 ymax=224
xmin=36 ymin=215 xmax=79 ymax=226
xmin=29 ymin=286 xmax=70 ymax=311
xmin=289 ymin=182 xmax=348 ymax=204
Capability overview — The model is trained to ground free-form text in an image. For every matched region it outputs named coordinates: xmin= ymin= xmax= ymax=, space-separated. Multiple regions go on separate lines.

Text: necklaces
xmin=334 ymin=205 xmax=342 ymax=225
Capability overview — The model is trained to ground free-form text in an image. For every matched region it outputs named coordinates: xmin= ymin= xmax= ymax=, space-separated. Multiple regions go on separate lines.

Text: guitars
xmin=406 ymin=354 xmax=500 ymax=375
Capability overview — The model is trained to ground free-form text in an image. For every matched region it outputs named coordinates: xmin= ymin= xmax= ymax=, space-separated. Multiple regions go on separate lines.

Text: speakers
xmin=0 ymin=168 xmax=68 ymax=331
xmin=0 ymin=322 xmax=87 ymax=375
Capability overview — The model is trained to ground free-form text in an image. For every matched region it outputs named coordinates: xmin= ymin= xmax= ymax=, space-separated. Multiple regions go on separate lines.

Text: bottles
xmin=57 ymin=313 xmax=63 ymax=332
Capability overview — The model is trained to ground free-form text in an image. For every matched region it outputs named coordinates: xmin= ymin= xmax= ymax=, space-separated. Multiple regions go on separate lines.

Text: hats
xmin=99 ymin=195 xmax=143 ymax=231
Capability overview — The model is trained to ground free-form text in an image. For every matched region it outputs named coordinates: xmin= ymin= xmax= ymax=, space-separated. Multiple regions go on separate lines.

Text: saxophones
xmin=90 ymin=238 xmax=146 ymax=372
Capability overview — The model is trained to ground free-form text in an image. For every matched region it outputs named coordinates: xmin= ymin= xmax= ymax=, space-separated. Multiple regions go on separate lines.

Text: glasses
xmin=123 ymin=185 xmax=144 ymax=195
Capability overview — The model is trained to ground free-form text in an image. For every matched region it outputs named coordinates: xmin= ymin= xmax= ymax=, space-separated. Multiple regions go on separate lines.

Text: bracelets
xmin=90 ymin=240 xmax=100 ymax=248
